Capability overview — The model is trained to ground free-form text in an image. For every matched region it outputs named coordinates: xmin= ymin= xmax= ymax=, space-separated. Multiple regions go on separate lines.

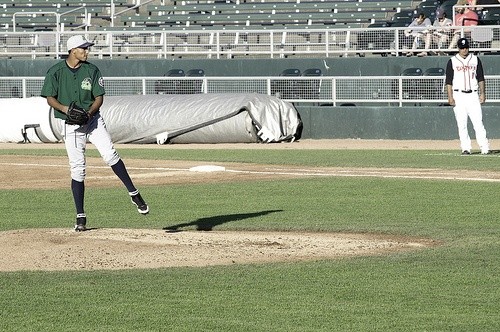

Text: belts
xmin=454 ymin=89 xmax=477 ymax=93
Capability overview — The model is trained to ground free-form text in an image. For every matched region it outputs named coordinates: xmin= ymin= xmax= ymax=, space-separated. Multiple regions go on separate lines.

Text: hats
xmin=413 ymin=9 xmax=425 ymax=18
xmin=436 ymin=9 xmax=446 ymax=18
xmin=457 ymin=38 xmax=469 ymax=49
xmin=67 ymin=34 xmax=95 ymax=52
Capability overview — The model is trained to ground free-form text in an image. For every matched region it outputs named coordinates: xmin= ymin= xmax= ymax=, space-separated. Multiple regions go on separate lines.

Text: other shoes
xmin=462 ymin=150 xmax=470 ymax=154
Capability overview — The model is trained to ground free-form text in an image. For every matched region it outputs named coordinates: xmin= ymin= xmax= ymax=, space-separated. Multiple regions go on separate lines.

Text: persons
xmin=40 ymin=34 xmax=149 ymax=232
xmin=404 ymin=0 xmax=478 ymax=57
xmin=445 ymin=37 xmax=491 ymax=155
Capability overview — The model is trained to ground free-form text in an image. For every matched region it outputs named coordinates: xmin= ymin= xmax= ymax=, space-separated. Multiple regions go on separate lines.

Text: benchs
xmin=0 ymin=0 xmax=420 ymax=58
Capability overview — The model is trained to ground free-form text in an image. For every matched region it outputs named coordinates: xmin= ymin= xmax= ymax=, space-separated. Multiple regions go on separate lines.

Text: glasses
xmin=462 ymin=4 xmax=469 ymax=6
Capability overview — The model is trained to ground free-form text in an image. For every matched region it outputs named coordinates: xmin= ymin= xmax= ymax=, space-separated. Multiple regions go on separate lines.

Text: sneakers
xmin=129 ymin=189 xmax=149 ymax=214
xmin=75 ymin=214 xmax=86 ymax=231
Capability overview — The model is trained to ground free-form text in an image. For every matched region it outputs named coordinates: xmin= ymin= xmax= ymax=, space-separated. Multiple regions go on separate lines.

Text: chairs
xmin=271 ymin=68 xmax=323 ymax=106
xmin=155 ymin=68 xmax=204 ymax=94
xmin=392 ymin=67 xmax=446 ymax=106
xmin=358 ymin=0 xmax=500 ymax=55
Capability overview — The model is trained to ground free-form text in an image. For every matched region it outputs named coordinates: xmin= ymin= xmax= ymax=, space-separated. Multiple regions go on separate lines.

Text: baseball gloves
xmin=64 ymin=101 xmax=90 ymax=126
